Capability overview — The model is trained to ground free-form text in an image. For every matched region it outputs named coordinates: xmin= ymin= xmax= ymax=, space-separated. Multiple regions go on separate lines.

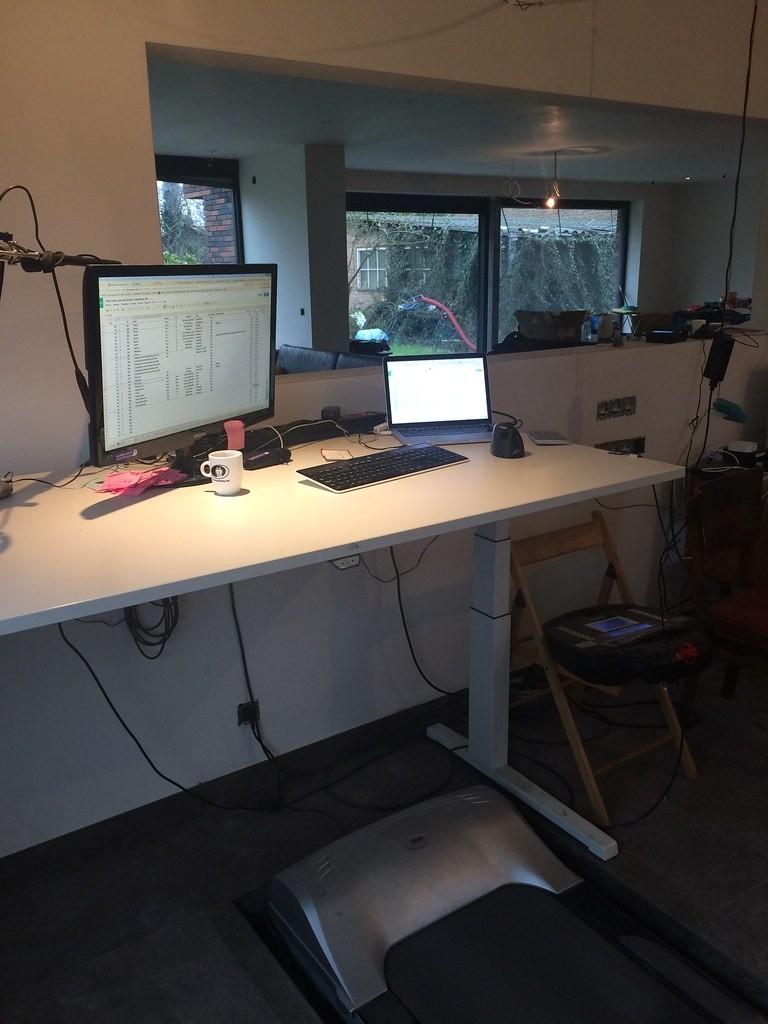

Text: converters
xmin=703 ymin=333 xmax=735 ymax=391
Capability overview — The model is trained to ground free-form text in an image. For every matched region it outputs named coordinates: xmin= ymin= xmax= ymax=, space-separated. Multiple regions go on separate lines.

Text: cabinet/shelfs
xmin=694 ymin=469 xmax=768 ymax=591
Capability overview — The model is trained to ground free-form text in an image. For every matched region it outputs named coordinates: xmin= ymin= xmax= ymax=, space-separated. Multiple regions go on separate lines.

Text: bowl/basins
xmin=704 ymin=301 xmax=721 ymax=308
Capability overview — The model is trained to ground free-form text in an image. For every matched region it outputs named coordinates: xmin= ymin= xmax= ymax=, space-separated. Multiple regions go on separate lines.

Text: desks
xmin=1 ymin=430 xmax=685 ymax=860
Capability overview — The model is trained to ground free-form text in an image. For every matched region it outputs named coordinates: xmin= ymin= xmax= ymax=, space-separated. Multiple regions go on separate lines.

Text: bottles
xmin=580 ymin=321 xmax=592 ymax=339
xmin=641 ymin=332 xmax=646 ymax=343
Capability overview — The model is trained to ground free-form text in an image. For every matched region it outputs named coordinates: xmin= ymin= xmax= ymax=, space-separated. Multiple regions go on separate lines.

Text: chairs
xmin=508 ymin=509 xmax=700 ymax=829
xmin=691 ymin=468 xmax=768 ymax=698
xmin=276 ymin=345 xmax=381 ymax=374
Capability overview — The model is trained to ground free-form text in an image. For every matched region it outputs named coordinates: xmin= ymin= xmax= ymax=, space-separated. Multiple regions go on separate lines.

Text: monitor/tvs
xmin=82 ymin=263 xmax=283 ymax=487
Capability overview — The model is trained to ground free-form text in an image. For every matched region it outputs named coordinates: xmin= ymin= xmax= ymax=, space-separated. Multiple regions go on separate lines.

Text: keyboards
xmin=297 ymin=442 xmax=469 ymax=493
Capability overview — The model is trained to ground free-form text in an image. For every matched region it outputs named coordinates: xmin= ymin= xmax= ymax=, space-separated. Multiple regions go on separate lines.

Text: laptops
xmin=382 ymin=352 xmax=495 ymax=447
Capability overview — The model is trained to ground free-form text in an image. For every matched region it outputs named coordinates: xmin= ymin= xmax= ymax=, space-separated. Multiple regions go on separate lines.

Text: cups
xmin=709 ymin=323 xmax=722 ymax=331
xmin=200 ymin=450 xmax=243 ymax=496
xmin=598 ymin=315 xmax=613 ymax=338
xmin=692 ymin=320 xmax=706 ymax=333
